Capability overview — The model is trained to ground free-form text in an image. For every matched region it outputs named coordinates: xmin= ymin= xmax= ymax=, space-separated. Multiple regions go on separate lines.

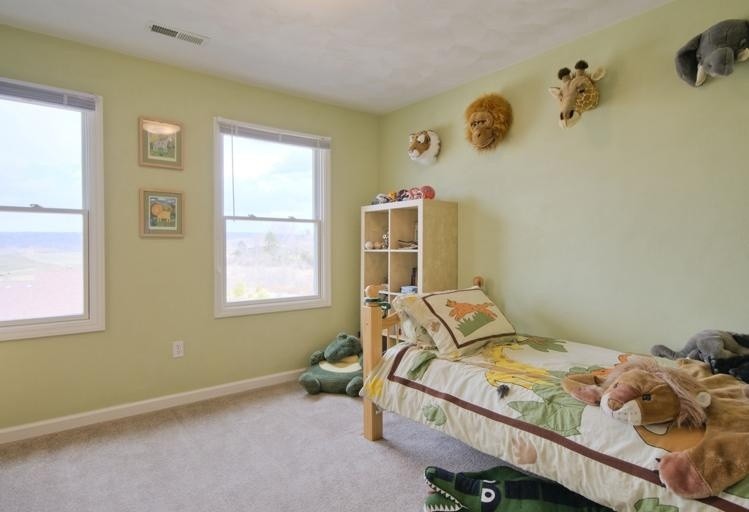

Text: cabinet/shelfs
xmin=360 ymin=198 xmax=459 ymax=353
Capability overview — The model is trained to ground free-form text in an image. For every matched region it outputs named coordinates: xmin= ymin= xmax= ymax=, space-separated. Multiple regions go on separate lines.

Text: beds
xmin=362 ymin=280 xmax=749 ymax=511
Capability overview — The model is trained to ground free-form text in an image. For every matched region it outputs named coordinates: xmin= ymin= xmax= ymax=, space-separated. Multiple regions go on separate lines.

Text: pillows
xmin=390 ymin=283 xmax=519 ymax=364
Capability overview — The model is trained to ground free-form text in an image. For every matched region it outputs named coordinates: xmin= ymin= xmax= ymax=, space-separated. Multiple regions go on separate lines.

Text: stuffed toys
xmin=558 ymin=351 xmax=749 ymax=500
xmin=371 ymin=185 xmax=435 ymax=205
xmin=297 ymin=330 xmax=363 ymax=398
xmin=421 ymin=463 xmax=615 ymax=511
xmin=674 ymin=21 xmax=749 ymax=88
xmin=465 ymin=93 xmax=512 ymax=150
xmin=407 ymin=131 xmax=440 ymax=166
xmin=547 ymin=61 xmax=606 ymax=130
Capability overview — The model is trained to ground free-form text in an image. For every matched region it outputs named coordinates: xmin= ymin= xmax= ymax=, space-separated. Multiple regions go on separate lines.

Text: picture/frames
xmin=137 ymin=114 xmax=187 ymax=239
